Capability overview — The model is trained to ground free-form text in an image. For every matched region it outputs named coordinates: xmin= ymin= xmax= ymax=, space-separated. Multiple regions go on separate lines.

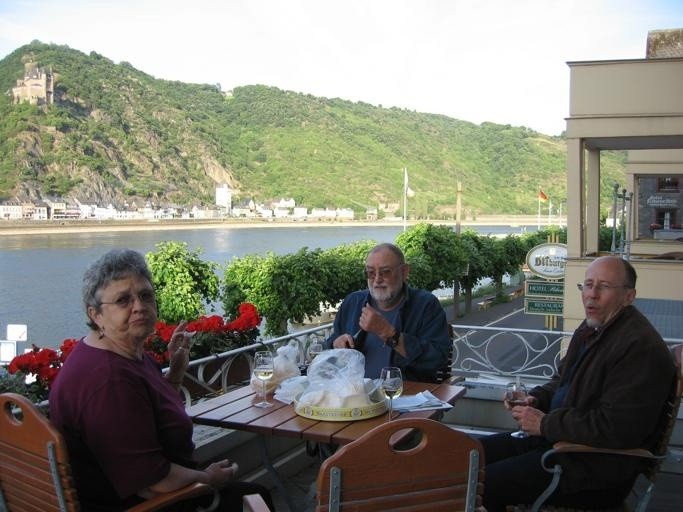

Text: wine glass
xmin=253 ymin=351 xmax=275 ymax=409
xmin=508 ymin=382 xmax=529 ymax=438
xmin=379 ymin=367 xmax=403 ymax=424
xmin=309 ymin=344 xmax=322 ymax=363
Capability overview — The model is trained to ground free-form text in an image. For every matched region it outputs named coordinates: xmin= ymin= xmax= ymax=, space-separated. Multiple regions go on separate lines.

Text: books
xmin=385 ymin=389 xmax=454 ymax=413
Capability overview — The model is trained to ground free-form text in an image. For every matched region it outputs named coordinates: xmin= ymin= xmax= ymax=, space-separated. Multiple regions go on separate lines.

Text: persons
xmin=315 ymin=241 xmax=451 ymax=386
xmin=46 ymin=246 xmax=274 ymax=511
xmin=475 ymin=255 xmax=678 ymax=511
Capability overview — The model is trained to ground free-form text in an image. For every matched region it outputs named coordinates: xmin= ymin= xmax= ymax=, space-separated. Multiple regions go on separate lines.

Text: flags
xmin=404 ymin=169 xmax=416 ymax=199
xmin=538 ymin=188 xmax=549 ymax=203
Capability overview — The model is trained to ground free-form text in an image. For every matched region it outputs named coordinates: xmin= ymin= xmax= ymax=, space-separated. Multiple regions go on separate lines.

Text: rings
xmin=178 ymin=346 xmax=186 ymax=352
xmin=169 ymin=337 xmax=177 ymax=344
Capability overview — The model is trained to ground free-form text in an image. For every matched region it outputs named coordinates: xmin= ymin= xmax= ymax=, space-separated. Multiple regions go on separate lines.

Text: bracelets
xmin=163 ymin=374 xmax=183 ymax=384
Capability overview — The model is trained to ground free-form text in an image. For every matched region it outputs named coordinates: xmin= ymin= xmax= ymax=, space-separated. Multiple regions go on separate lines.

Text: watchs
xmin=385 ymin=329 xmax=401 ymax=349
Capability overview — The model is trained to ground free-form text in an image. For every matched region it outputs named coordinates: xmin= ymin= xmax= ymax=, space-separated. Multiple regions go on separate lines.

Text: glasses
xmin=364 ymin=268 xmax=394 ymax=277
xmin=583 ymin=281 xmax=625 ymax=290
xmin=100 ymin=291 xmax=155 ymax=306
